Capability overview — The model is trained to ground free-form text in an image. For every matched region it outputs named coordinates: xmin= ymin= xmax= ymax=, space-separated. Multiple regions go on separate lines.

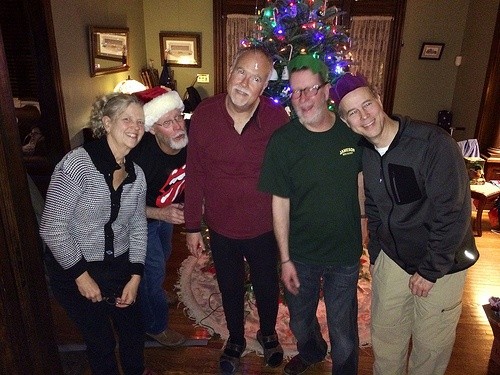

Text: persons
xmin=38 ymin=44 xmax=480 ymax=375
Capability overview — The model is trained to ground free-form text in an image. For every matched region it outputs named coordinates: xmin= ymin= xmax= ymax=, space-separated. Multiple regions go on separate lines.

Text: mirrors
xmin=89 ymin=26 xmax=129 ymax=78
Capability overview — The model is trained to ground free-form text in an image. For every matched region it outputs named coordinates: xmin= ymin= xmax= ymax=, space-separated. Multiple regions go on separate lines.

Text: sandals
xmin=219 ymin=337 xmax=246 ymax=375
xmin=257 ymin=329 xmax=284 ymax=368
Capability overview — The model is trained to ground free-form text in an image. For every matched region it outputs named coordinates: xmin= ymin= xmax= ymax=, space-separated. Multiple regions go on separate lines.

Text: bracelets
xmin=280 ymin=259 xmax=291 ymax=265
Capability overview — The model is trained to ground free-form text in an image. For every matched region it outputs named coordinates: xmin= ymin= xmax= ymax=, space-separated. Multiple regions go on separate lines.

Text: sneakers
xmin=146 ymin=328 xmax=185 ymax=346
xmin=283 ymin=354 xmax=309 ymax=375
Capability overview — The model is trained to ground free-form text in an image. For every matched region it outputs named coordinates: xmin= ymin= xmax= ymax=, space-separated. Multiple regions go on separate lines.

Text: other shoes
xmin=21 ymin=128 xmax=46 ymax=156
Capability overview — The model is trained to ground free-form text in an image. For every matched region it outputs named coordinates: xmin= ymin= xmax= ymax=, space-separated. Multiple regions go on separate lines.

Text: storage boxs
xmin=463 ymin=156 xmax=485 ymax=180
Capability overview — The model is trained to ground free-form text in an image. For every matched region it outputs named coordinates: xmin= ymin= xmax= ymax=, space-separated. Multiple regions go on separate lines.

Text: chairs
xmin=457 ymin=138 xmax=500 ymax=237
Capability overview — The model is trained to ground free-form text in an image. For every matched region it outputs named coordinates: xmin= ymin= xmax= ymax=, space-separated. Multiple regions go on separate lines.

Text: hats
xmin=131 ymin=85 xmax=184 ymax=132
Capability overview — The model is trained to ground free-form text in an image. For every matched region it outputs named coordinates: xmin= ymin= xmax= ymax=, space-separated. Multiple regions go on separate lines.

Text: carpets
xmin=178 ymin=241 xmax=373 ymax=362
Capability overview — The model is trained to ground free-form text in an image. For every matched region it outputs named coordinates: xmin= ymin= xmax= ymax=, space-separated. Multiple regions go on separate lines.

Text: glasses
xmin=287 ymin=81 xmax=328 ymax=101
xmin=155 ymin=115 xmax=183 ymax=128
xmin=101 ymin=295 xmax=132 ymax=306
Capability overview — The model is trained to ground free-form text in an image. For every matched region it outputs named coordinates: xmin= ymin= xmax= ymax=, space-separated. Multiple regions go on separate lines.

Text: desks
xmin=479 ymin=153 xmax=500 ymax=182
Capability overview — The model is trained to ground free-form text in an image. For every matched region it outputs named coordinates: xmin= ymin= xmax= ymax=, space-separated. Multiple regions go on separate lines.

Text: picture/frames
xmin=418 ymin=43 xmax=445 ymax=60
xmin=94 ymin=33 xmax=123 ymax=61
xmin=159 ymin=33 xmax=202 ymax=68
